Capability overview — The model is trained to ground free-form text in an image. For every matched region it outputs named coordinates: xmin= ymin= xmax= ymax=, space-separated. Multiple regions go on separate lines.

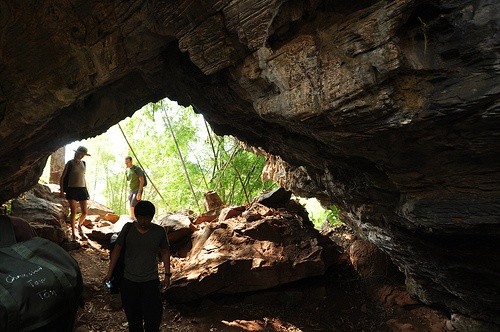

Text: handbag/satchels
xmin=110 ymin=241 xmax=124 ymax=294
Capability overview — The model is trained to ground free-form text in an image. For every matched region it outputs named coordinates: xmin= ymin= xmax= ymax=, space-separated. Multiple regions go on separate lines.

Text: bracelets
xmin=60 ymin=188 xmax=64 ymax=191
xmin=165 ymin=273 xmax=172 ymax=277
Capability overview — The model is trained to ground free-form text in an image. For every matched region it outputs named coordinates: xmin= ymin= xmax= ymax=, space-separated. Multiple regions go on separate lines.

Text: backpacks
xmin=135 ymin=168 xmax=147 ymax=187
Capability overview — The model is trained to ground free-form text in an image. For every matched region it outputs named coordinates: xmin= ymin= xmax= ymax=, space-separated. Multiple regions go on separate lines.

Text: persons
xmin=125 ymin=157 xmax=144 ymax=220
xmin=59 ymin=147 xmax=90 ymax=240
xmin=0 ymin=214 xmax=38 ymax=248
xmin=101 ymin=200 xmax=172 ymax=332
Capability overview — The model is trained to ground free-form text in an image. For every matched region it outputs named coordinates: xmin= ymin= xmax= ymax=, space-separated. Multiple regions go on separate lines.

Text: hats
xmin=76 ymin=148 xmax=89 ymax=156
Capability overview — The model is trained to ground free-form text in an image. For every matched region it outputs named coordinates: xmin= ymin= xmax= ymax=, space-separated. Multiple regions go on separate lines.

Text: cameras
xmin=105 ymin=281 xmax=112 ymax=289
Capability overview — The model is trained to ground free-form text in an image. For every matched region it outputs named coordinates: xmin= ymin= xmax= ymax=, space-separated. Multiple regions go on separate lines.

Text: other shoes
xmin=75 ymin=224 xmax=83 ymax=236
xmin=71 ymin=235 xmax=75 ymax=241
